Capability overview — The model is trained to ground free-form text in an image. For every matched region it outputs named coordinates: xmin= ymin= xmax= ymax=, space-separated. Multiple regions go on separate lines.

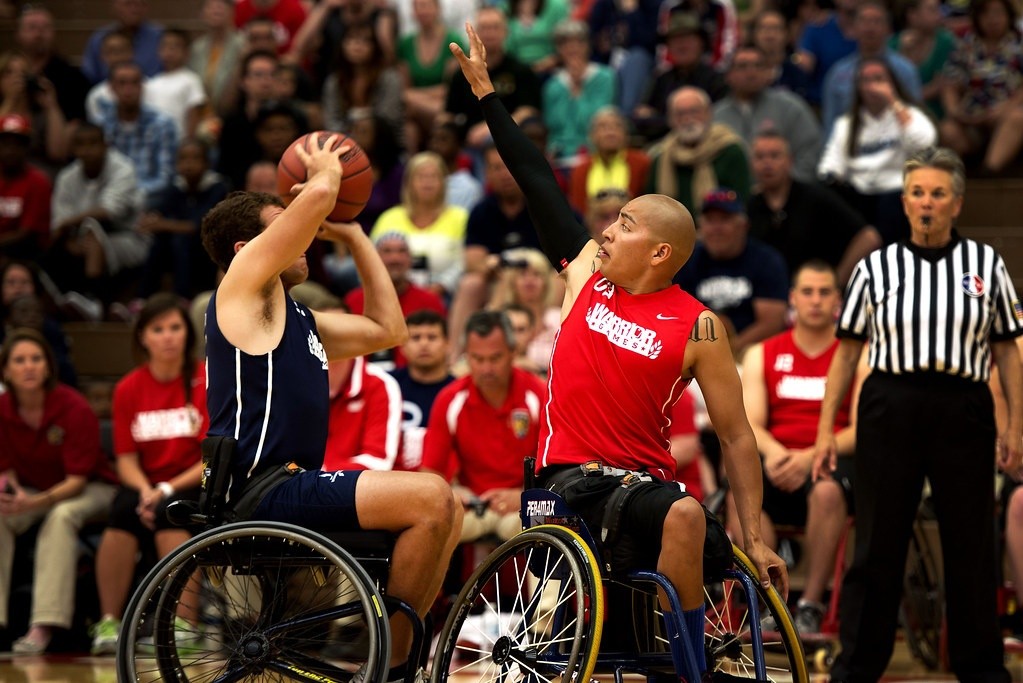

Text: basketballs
xmin=277 ymin=130 xmax=374 ymax=223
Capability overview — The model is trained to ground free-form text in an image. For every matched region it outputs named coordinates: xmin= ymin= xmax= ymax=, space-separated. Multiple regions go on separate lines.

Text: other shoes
xmin=793 ymin=600 xmax=822 ymax=632
xmin=12 ymin=631 xmax=55 ymax=653
xmin=746 ymin=605 xmax=788 ymax=633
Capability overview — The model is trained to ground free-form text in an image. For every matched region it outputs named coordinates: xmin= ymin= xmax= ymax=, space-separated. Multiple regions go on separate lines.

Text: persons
xmin=812 ymin=147 xmax=1023 ymax=683
xmin=448 ymin=21 xmax=789 ymax=683
xmin=0 ymin=0 xmax=1023 ymax=654
xmin=200 ymin=130 xmax=463 ymax=683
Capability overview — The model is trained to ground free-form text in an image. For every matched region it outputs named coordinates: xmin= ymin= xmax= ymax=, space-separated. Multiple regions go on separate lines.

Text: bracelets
xmin=158 ymin=483 xmax=175 ymax=498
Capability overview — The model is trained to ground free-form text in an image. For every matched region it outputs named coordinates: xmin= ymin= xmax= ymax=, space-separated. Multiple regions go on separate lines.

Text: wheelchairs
xmin=696 ymin=506 xmax=951 ymax=675
xmin=427 ymin=457 xmax=810 ymax=683
xmin=114 ymin=437 xmax=437 ymax=683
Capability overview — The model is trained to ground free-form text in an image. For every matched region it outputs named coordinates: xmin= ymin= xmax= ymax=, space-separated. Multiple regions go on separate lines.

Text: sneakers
xmin=135 ymin=616 xmax=208 ymax=653
xmin=349 ymin=658 xmax=431 ymax=683
xmin=90 ymin=615 xmax=122 ymax=655
